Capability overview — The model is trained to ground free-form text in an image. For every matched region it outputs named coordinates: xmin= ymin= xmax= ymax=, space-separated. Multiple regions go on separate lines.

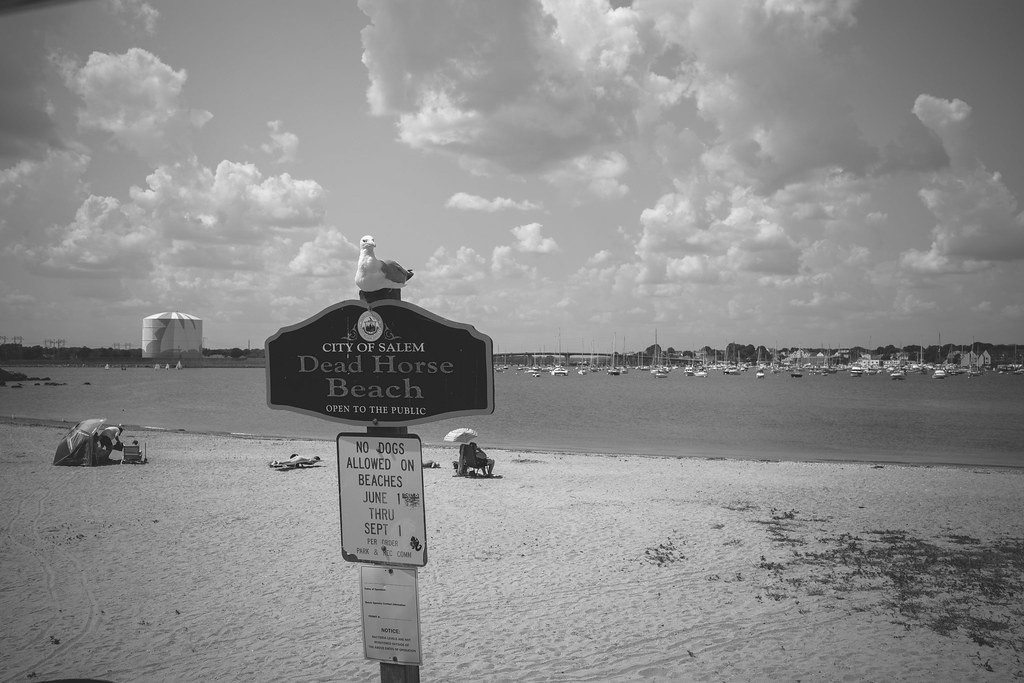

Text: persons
xmin=132 ymin=440 xmax=138 ymax=446
xmin=274 ymin=455 xmax=320 ymax=467
xmin=459 ymin=442 xmax=495 ymax=478
xmin=99 ymin=426 xmax=123 ymax=462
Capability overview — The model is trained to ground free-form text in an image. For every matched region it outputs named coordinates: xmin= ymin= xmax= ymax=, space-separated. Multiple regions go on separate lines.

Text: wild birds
xmin=355 ymin=235 xmax=414 ymax=292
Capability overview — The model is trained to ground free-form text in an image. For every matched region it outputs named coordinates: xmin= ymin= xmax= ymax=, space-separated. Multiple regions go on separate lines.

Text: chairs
xmin=120 ymin=444 xmax=142 ymax=465
xmin=458 ymin=444 xmax=490 ymax=477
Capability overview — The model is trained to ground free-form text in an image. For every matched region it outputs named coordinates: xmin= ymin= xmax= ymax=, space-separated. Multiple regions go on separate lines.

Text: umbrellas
xmin=53 ymin=418 xmax=107 ymax=466
xmin=443 ymin=428 xmax=478 ymax=477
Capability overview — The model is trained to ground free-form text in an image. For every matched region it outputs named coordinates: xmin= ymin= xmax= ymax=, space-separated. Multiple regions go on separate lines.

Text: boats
xmin=154 ymin=360 xmax=183 ymax=370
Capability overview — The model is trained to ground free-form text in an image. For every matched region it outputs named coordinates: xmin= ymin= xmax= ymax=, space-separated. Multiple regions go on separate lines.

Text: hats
xmin=118 ymin=424 xmax=125 ymax=430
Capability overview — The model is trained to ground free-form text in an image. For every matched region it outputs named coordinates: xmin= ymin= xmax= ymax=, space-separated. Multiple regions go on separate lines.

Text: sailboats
xmin=494 ymin=332 xmax=1024 ymax=380
